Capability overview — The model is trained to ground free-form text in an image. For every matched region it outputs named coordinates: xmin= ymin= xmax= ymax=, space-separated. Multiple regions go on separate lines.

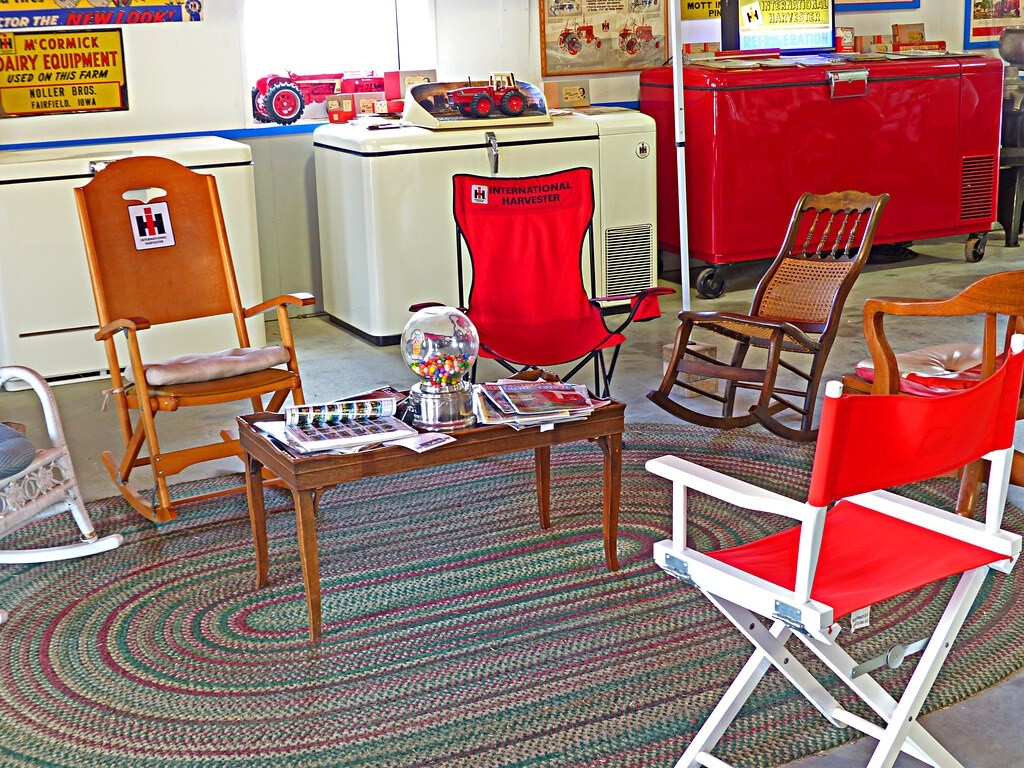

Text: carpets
xmin=0 ymin=422 xmax=1024 ymax=768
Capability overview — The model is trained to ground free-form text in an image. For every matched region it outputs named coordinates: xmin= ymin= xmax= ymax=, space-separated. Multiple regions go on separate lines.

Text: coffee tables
xmin=235 ymin=372 xmax=628 ymax=644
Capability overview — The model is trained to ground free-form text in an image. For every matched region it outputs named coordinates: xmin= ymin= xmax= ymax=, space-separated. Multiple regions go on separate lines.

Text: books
xmin=248 ymin=375 xmax=612 ymax=455
xmin=681 ymin=23 xmax=987 ymax=68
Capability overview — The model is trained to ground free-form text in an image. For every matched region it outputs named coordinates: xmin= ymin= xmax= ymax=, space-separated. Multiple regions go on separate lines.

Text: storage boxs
xmin=892 ymin=22 xmax=926 ymax=44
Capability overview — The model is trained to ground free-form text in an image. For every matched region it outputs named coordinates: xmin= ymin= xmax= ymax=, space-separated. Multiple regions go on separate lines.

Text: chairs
xmin=73 ymin=156 xmax=306 ymax=525
xmin=0 ymin=365 xmax=125 ymax=622
xmin=646 ymin=190 xmax=891 ymax=442
xmin=642 ymin=334 xmax=1024 ymax=768
xmin=408 ymin=165 xmax=675 ymax=449
xmin=837 ymin=270 xmax=1024 ymax=521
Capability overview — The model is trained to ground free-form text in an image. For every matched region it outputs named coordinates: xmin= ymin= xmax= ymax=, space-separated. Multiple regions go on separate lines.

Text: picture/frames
xmin=538 ymin=0 xmax=669 ymax=77
xmin=964 ymin=0 xmax=1024 ymax=50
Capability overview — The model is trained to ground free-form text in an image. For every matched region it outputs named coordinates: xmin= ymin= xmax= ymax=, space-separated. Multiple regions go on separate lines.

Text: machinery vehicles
xmin=252 ymin=71 xmax=385 ymax=126
xmin=619 ymin=15 xmax=660 ymax=54
xmin=558 ymin=13 xmax=603 ymax=55
xmin=447 ymin=71 xmax=529 ymax=117
